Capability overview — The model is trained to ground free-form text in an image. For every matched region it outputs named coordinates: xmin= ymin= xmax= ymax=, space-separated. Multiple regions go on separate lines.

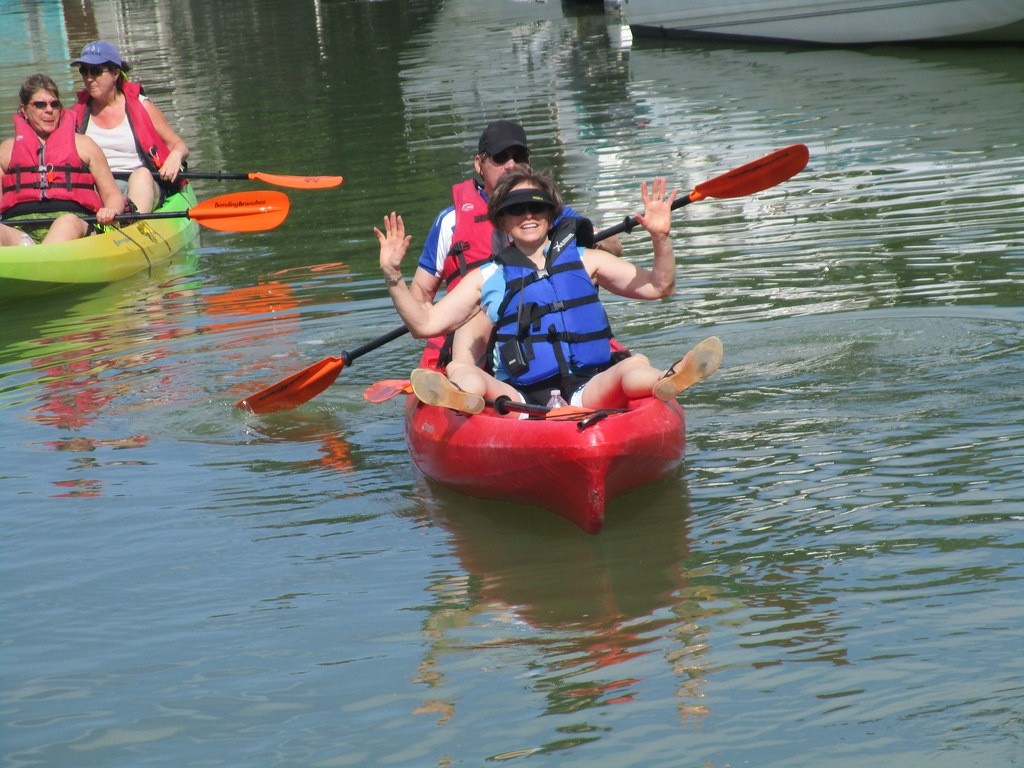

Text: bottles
xmin=20 ymin=233 xmax=33 ymax=246
xmin=546 ymin=390 xmax=568 ymax=411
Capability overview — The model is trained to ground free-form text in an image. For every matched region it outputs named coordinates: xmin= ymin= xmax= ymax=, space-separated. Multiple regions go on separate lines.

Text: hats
xmin=478 ymin=119 xmax=532 ymax=156
xmin=70 ymin=41 xmax=122 ymax=66
xmin=489 ymin=189 xmax=556 ymax=220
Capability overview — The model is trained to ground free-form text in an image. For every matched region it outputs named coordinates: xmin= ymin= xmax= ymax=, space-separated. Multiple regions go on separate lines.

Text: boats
xmin=0 ymin=168 xmax=201 ymax=299
xmin=404 ymin=337 xmax=686 ymax=537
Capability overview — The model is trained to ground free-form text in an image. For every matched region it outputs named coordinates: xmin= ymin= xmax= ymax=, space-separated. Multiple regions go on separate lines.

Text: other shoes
xmin=119 ymin=197 xmax=141 ymax=227
xmin=650 ymin=337 xmax=723 ymax=404
xmin=411 ymin=366 xmax=487 ymax=414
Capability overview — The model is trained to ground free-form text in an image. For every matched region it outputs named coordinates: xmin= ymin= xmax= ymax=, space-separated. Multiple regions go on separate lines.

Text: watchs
xmin=384 ymin=276 xmax=403 ymax=286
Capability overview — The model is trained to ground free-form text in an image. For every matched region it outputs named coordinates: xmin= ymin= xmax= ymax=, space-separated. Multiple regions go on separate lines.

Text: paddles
xmin=0 ymin=190 xmax=291 ymax=233
xmin=112 ymin=170 xmax=343 ymax=189
xmin=236 ymin=142 xmax=811 ymax=412
xmin=363 ymin=378 xmax=596 ymax=419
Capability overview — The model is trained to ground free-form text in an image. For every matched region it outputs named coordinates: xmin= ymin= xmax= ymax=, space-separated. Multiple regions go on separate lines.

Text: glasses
xmin=79 ymin=66 xmax=111 ymax=77
xmin=492 ymin=151 xmax=529 ymax=164
xmin=506 ymin=202 xmax=547 ymax=216
xmin=31 ymin=100 xmax=61 ymax=108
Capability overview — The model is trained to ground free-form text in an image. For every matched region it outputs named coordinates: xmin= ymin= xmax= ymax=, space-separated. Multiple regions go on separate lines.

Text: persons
xmin=407 ymin=120 xmax=624 ymax=362
xmin=373 ymin=168 xmax=723 ymax=414
xmin=71 ymin=40 xmax=189 ymax=212
xmin=0 ymin=73 xmax=123 ymax=248
